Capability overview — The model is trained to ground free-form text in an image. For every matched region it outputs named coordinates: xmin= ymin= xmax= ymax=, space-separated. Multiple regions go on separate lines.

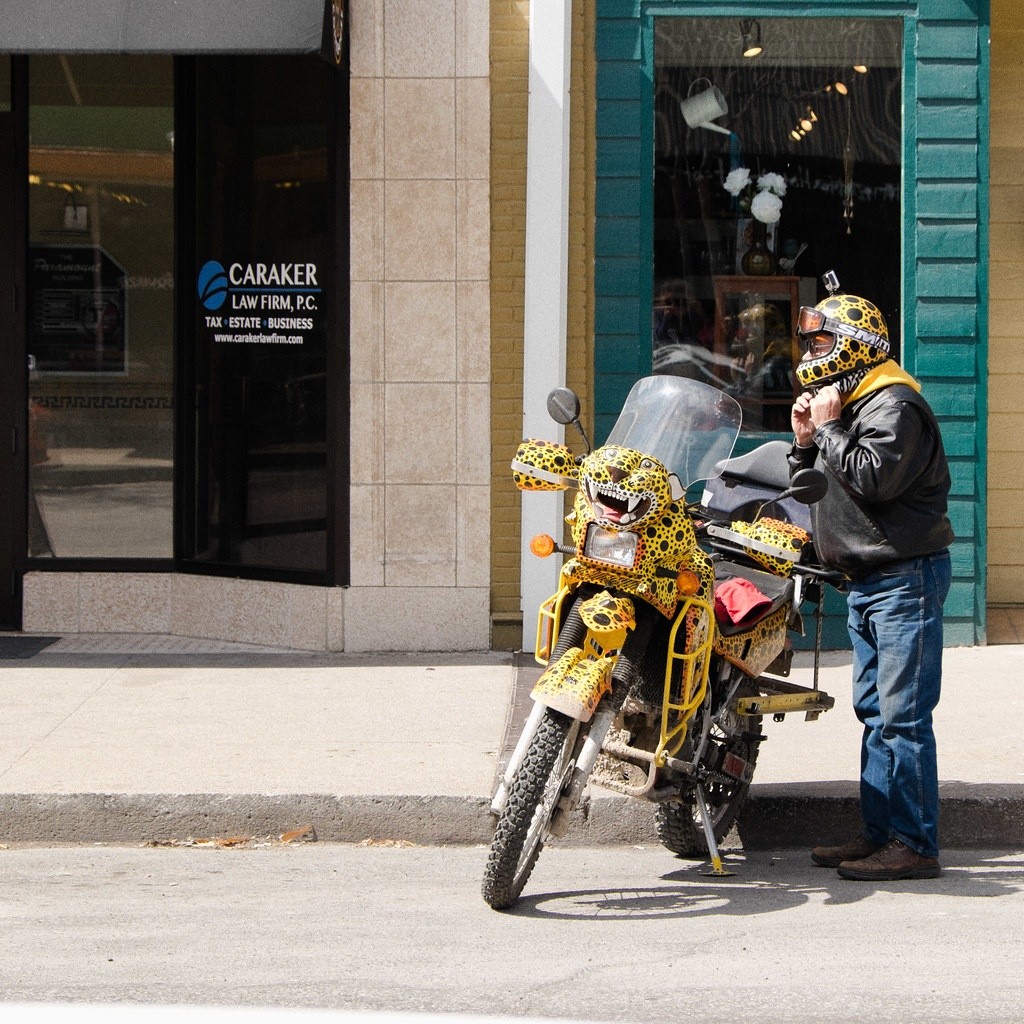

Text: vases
xmin=741 ymin=218 xmax=778 ymax=275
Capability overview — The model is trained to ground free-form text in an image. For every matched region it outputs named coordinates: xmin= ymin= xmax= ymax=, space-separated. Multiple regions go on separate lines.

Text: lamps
xmin=835 ymin=79 xmax=849 ymax=95
xmin=738 ymin=16 xmax=763 ymax=59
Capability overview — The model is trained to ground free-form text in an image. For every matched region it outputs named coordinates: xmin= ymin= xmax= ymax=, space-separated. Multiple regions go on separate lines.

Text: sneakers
xmin=835 ymin=840 xmax=941 ymax=878
xmin=812 ymin=834 xmax=880 ymax=866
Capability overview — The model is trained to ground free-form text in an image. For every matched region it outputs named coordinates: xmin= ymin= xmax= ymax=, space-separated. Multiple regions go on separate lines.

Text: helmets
xmin=797 ymin=293 xmax=889 ymax=388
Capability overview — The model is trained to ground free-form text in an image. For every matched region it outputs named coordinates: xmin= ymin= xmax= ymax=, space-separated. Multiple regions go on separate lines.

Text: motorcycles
xmin=478 ymin=374 xmax=856 ymax=914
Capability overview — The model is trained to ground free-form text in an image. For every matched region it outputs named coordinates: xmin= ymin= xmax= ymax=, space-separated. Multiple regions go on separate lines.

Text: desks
xmin=711 ymin=274 xmax=804 ymax=406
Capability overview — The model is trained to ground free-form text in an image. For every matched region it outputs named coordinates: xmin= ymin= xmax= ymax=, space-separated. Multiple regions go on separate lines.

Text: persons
xmin=786 ymin=293 xmax=955 ymax=881
xmin=653 ymin=277 xmax=696 ymax=349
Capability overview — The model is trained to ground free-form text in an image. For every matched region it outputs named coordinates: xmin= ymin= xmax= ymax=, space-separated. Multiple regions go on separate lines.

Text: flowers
xmin=724 ymin=166 xmax=788 ymax=225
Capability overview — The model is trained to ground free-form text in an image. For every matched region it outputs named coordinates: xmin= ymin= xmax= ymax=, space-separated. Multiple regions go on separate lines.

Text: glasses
xmin=808 ymin=341 xmax=833 ymax=353
xmin=793 ymin=306 xmax=826 ymax=336
